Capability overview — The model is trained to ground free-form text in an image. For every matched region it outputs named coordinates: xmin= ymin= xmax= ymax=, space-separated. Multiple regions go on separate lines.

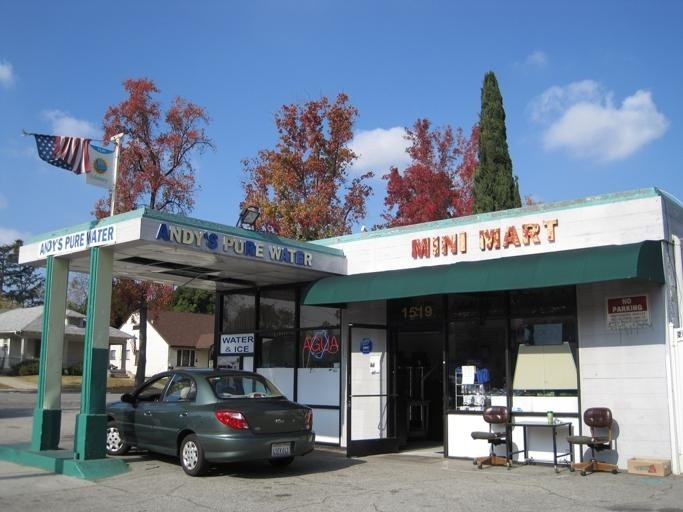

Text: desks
xmin=507 ymin=418 xmax=572 ymax=475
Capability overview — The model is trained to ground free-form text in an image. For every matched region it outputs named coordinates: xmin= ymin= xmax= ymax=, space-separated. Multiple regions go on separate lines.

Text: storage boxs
xmin=626 ymin=456 xmax=671 ymax=478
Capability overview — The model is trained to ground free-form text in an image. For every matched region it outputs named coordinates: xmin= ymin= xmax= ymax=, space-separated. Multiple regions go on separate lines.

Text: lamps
xmin=236 ymin=205 xmax=262 ymax=234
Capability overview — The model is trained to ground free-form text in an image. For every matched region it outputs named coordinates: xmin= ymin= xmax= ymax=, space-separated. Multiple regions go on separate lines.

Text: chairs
xmin=565 ymin=407 xmax=619 ymax=476
xmin=470 ymin=406 xmax=510 ymax=471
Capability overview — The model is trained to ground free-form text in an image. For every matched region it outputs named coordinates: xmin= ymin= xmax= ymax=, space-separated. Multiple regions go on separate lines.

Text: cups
xmin=546 ymin=411 xmax=553 ymax=424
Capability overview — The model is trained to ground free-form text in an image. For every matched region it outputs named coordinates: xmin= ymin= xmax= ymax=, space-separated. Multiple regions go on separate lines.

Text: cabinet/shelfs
xmin=454 ymin=365 xmax=491 ymax=412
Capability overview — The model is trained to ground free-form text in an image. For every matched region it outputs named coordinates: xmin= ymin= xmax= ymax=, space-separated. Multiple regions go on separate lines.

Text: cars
xmin=103 ymin=366 xmax=316 ymax=476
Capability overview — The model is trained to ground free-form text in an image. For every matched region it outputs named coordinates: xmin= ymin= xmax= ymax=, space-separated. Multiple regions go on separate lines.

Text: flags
xmin=34 ymin=134 xmax=92 ymax=174
